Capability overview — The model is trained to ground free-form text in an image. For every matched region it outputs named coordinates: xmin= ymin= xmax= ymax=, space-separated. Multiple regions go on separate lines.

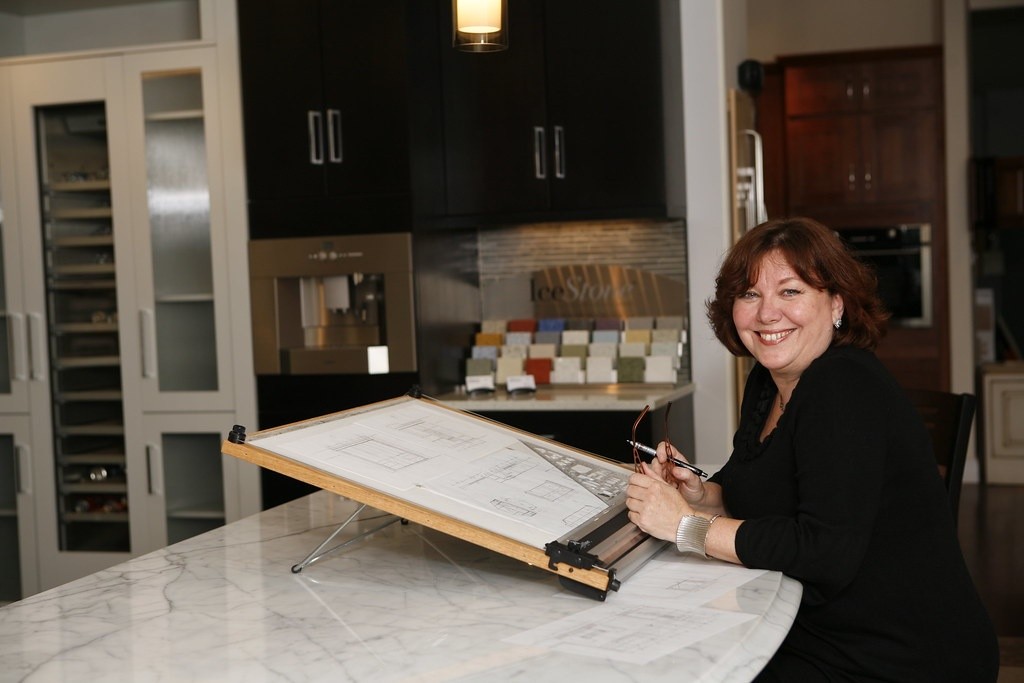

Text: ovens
xmin=828 ymin=222 xmax=933 ymax=327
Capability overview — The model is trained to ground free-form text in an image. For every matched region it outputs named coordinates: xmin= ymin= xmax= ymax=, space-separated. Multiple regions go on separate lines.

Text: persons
xmin=627 ymin=217 xmax=1000 ymax=683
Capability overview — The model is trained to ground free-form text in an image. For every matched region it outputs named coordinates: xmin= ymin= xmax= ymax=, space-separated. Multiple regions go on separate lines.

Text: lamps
xmin=448 ymin=0 xmax=512 ymax=55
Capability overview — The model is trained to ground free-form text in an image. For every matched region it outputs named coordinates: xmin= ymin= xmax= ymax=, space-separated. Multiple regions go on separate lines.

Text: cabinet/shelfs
xmin=233 ymin=0 xmax=670 ymax=238
xmin=0 ymin=52 xmax=266 ymax=605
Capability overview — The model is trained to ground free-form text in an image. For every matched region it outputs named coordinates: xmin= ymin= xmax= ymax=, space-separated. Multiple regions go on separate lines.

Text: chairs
xmin=889 ymin=383 xmax=1002 ymax=683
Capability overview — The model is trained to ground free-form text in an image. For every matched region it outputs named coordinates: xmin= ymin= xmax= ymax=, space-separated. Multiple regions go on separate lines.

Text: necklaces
xmin=778 ymin=392 xmax=786 ymax=412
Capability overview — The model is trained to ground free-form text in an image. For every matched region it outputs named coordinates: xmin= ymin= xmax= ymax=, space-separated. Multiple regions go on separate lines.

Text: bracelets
xmin=677 ymin=510 xmax=723 ymax=560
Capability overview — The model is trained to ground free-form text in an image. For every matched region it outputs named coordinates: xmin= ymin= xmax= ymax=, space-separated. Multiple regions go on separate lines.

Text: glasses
xmin=631 ymin=400 xmax=680 ymax=490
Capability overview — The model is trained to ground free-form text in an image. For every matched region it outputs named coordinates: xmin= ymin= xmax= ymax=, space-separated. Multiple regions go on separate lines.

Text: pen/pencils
xmin=626 ymin=439 xmax=708 ymax=477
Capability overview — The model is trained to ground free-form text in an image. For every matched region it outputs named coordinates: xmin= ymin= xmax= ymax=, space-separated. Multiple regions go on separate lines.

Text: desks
xmin=2 ymin=492 xmax=805 ymax=683
